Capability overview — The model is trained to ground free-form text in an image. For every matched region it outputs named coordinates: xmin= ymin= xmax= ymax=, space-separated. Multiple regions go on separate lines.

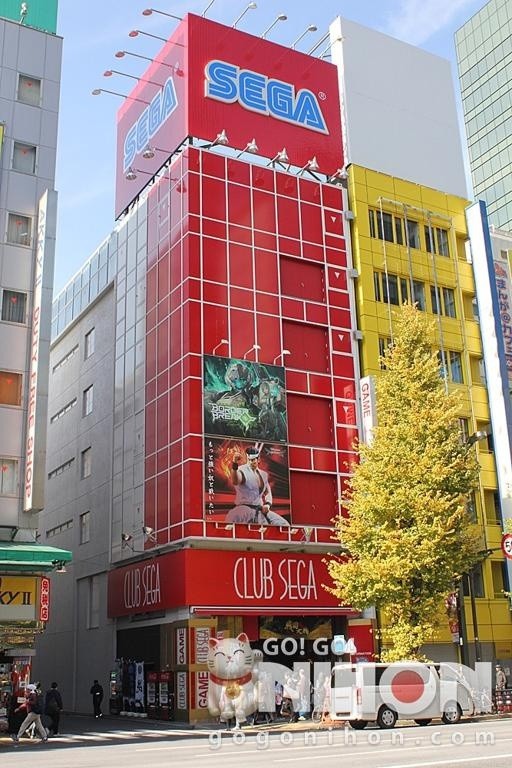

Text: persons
xmin=90 ymin=680 xmax=104 ymax=720
xmin=493 ymin=665 xmax=507 ymax=690
xmin=9 ymin=681 xmax=64 ymax=744
xmin=219 ymin=667 xmax=332 ymax=731
xmin=223 ymin=446 xmax=290 ymax=526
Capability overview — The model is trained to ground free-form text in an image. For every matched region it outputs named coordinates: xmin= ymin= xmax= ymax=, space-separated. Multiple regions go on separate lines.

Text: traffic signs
xmin=500 ymin=534 xmax=512 ymax=560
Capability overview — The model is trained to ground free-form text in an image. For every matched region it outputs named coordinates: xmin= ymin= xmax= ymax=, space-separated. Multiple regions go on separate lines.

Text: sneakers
xmin=25 ymin=729 xmax=37 ymax=739
xmin=42 ymin=729 xmax=59 ymax=744
xmin=9 ymin=734 xmax=18 ymax=742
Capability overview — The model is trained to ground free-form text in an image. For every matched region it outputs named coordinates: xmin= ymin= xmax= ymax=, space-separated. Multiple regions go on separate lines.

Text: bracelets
xmin=233 ymin=463 xmax=238 ymax=470
xmin=266 ymin=502 xmax=272 ymax=509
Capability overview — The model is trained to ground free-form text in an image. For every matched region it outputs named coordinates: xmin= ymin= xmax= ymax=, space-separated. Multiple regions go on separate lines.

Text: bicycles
xmin=311 ymin=687 xmax=325 ymax=723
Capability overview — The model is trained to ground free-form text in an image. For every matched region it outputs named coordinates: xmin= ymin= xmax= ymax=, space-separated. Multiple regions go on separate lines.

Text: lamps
xmin=214 ymin=520 xmax=299 ymax=535
xmin=208 ymin=129 xmax=349 ymax=184
xmin=233 ymin=1 xmax=319 ymax=51
xmin=126 ymin=142 xmax=175 ymax=186
xmin=122 ymin=525 xmax=160 ymax=552
xmin=91 ymin=7 xmax=182 ymax=101
xmin=212 ymin=338 xmax=290 ymax=364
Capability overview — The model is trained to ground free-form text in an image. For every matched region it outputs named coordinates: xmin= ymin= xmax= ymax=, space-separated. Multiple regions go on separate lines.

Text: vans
xmin=327 ymin=663 xmax=475 ymax=729
